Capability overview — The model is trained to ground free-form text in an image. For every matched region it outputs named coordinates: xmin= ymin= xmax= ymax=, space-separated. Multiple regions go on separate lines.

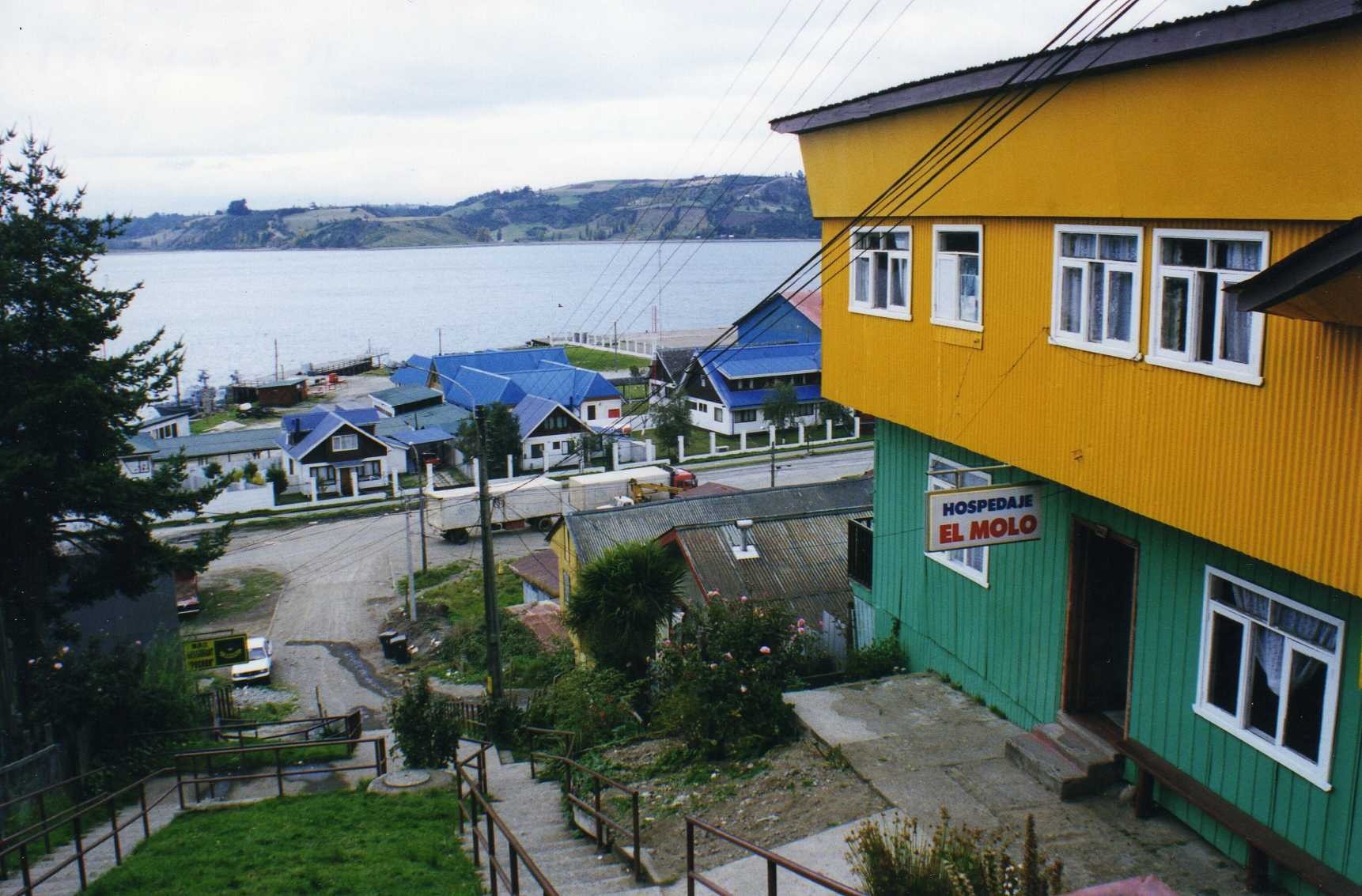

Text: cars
xmin=231 ymin=637 xmax=272 ymax=687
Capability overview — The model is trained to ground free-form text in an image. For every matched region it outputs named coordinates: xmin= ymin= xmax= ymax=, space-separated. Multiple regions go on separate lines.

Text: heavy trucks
xmin=424 ymin=463 xmax=697 ymax=546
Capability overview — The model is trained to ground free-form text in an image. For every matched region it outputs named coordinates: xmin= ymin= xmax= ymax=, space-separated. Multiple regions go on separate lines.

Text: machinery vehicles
xmin=595 ymin=478 xmax=685 ymax=510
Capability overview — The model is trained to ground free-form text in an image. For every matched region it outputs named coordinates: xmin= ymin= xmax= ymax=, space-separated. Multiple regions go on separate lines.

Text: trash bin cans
xmin=391 ymin=635 xmax=410 ymax=664
xmin=379 ymin=632 xmax=399 ymax=659
